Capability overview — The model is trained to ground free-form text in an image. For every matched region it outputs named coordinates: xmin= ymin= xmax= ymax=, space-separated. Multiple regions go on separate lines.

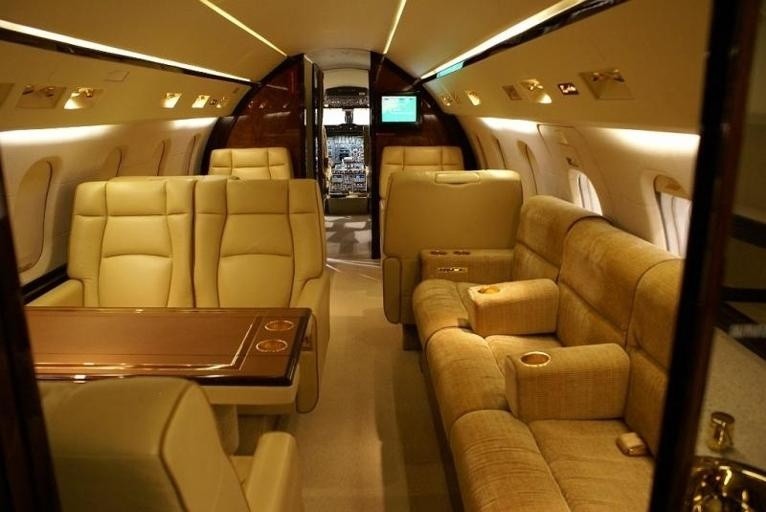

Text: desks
xmin=21 ymin=305 xmax=314 ymax=452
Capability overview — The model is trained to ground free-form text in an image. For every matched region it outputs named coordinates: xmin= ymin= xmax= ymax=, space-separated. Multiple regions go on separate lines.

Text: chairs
xmin=23 ymin=174 xmax=332 ymax=418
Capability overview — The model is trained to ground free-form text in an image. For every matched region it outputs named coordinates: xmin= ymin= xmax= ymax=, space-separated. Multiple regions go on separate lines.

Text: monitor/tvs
xmin=378 ymin=92 xmax=420 ymax=133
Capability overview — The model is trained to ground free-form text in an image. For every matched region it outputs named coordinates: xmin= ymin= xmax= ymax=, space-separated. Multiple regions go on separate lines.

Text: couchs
xmin=411 ymin=194 xmax=615 ymax=356
xmin=381 ymin=168 xmax=524 ymax=327
xmin=424 ymin=217 xmax=686 ymax=512
xmin=207 ymin=146 xmax=296 ymax=180
xmin=34 ymin=377 xmax=305 ymax=509
xmin=378 ymin=144 xmax=466 ymax=269
